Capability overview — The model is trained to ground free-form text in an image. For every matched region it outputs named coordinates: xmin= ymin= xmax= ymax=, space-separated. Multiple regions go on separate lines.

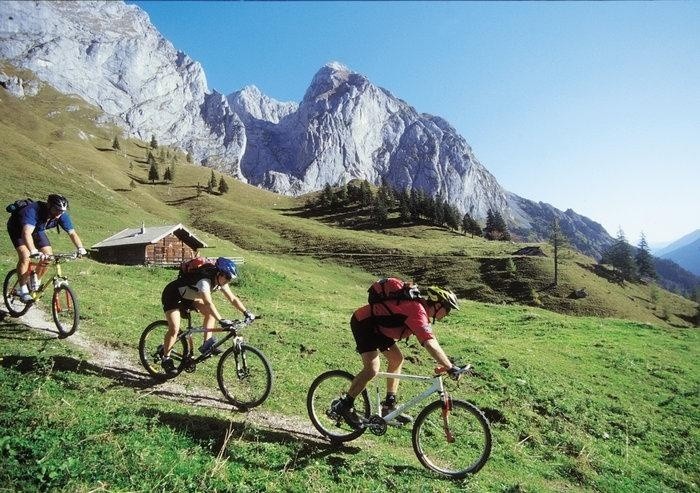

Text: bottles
xmin=198 ymin=336 xmax=216 ymax=353
xmin=31 ymin=270 xmax=38 ymax=289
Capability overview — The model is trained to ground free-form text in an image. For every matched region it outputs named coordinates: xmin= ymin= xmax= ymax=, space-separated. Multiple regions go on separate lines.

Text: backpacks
xmin=367 ymin=276 xmax=424 ymax=304
xmin=5 ymin=196 xmax=36 ymax=212
xmin=179 ymin=256 xmax=216 ymax=276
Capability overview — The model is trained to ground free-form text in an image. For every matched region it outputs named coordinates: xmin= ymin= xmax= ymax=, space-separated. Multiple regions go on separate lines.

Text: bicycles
xmin=4 ymin=248 xmax=100 ymax=336
xmin=138 ymin=302 xmax=273 ymax=408
xmin=306 ymin=345 xmax=494 ymax=480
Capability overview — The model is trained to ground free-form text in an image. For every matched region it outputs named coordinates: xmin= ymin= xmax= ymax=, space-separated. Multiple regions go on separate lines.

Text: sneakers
xmin=336 ymin=399 xmax=362 ymax=427
xmin=380 ymin=400 xmax=414 ymax=424
xmin=20 ymin=292 xmax=34 ymax=302
xmin=161 ymin=357 xmax=178 ymax=375
xmin=201 ymin=340 xmax=223 ymax=355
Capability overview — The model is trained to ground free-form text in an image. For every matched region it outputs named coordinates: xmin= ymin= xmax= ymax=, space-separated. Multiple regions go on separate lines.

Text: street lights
xmin=550 ymin=214 xmax=560 ymax=288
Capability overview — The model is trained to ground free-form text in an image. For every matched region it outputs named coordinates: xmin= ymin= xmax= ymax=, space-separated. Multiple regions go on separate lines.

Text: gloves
xmin=217 ymin=317 xmax=232 ymax=329
xmin=243 ymin=309 xmax=255 ymax=323
xmin=29 ymin=250 xmax=49 ymax=261
xmin=447 ymin=364 xmax=463 ymax=382
xmin=76 ymin=246 xmax=89 ymax=256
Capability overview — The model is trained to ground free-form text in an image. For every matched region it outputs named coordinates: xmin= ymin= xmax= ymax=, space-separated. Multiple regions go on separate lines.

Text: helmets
xmin=425 ymin=285 xmax=460 ymax=312
xmin=47 ymin=192 xmax=69 ymax=211
xmin=214 ymin=256 xmax=238 ymax=279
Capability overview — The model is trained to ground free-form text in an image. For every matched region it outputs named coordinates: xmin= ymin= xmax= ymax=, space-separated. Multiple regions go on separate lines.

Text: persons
xmin=161 ymin=257 xmax=255 ymax=377
xmin=331 ymin=278 xmax=461 ymax=432
xmin=4 ymin=193 xmax=87 ymax=305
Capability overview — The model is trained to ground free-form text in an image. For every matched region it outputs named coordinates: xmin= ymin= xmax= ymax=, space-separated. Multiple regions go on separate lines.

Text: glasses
xmin=442 ymin=303 xmax=451 ymax=314
